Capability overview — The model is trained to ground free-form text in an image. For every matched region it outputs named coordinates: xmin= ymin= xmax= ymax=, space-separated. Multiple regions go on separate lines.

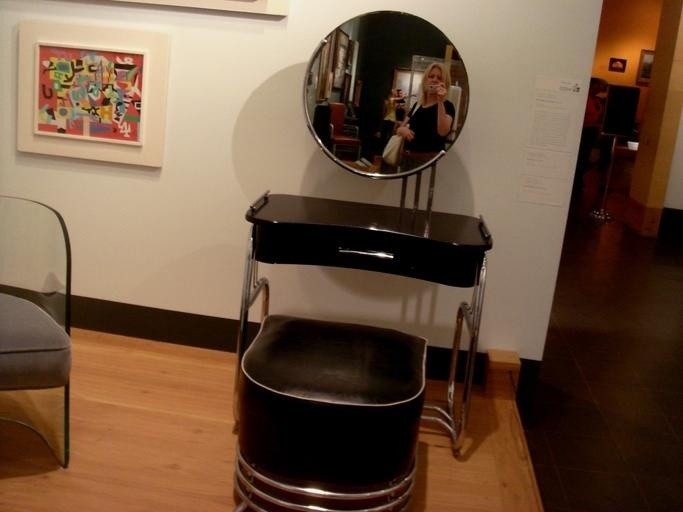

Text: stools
xmin=233 ymin=314 xmax=429 ymax=511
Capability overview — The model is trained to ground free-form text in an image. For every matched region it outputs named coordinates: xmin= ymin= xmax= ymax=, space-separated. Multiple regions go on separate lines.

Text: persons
xmin=572 ymin=78 xmax=608 ymax=208
xmin=356 ymin=88 xmax=418 ymax=173
xmin=396 ymin=62 xmax=455 ymax=173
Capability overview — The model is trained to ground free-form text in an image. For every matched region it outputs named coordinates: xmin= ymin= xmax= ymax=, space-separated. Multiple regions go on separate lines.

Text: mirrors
xmin=302 ymin=9 xmax=469 ymax=213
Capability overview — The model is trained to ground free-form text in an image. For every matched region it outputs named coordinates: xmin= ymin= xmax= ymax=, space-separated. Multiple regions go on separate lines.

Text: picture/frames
xmin=17 ymin=21 xmax=170 ymax=169
xmin=316 ymin=27 xmax=355 ymax=102
xmin=635 ymin=50 xmax=656 ymax=86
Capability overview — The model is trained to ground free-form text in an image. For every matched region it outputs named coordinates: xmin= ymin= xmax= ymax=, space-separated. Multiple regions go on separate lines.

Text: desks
xmin=231 ymin=189 xmax=492 ymax=459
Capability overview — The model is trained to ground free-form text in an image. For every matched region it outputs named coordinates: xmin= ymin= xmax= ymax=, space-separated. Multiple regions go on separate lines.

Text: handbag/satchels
xmin=380 ymin=100 xmax=421 ymax=165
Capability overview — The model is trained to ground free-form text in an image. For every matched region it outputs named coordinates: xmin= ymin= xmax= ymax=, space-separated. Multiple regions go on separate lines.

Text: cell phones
xmin=425 ymin=83 xmax=441 ymax=92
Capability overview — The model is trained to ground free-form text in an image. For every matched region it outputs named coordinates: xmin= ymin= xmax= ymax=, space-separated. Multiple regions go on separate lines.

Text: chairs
xmin=0 ymin=195 xmax=73 ymax=467
xmin=327 ymin=102 xmax=362 ymax=160
